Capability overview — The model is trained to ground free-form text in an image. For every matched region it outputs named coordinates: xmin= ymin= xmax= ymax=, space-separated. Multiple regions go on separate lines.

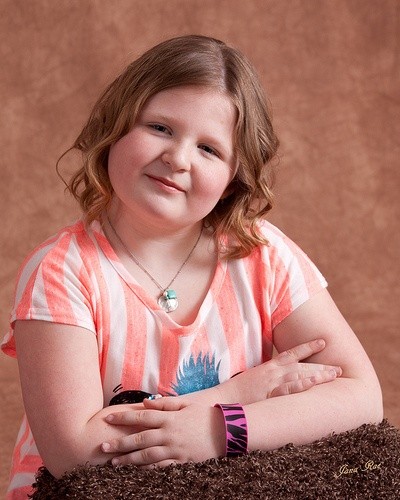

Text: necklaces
xmin=100 ymin=199 xmax=207 ymax=312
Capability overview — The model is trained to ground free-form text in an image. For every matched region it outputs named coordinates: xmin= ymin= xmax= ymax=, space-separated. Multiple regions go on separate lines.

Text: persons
xmin=1 ymin=33 xmax=384 ymax=479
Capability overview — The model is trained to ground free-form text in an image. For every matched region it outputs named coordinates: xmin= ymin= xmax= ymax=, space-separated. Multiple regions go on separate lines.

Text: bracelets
xmin=218 ymin=398 xmax=249 ymax=466
